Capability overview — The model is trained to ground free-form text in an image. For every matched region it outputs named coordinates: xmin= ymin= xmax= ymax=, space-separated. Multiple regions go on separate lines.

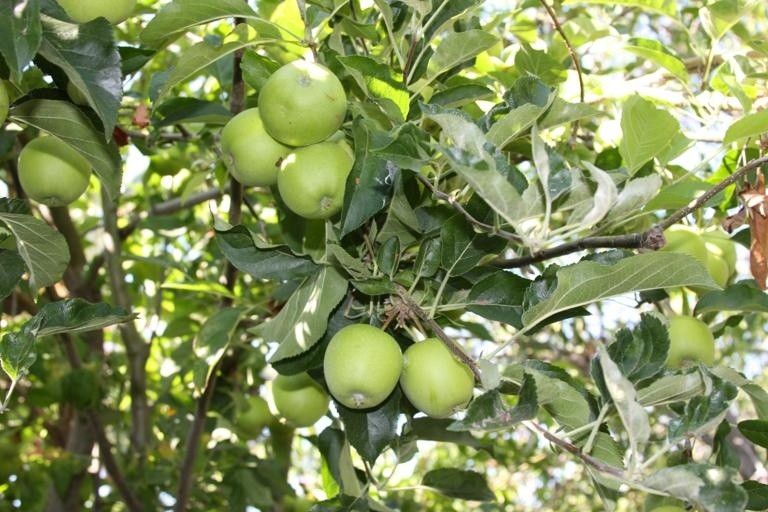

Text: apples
xmin=658 ymin=224 xmax=737 ymax=369
xmin=258 ymin=59 xmax=348 ymax=147
xmin=0 ymin=77 xmax=92 ymax=207
xmin=273 ymin=371 xmax=331 ymax=426
xmin=277 ymin=141 xmax=354 ymax=219
xmin=234 ymin=395 xmax=273 ymax=435
xmin=219 ymin=107 xmax=296 ymax=187
xmin=399 ymin=337 xmax=476 ymax=420
xmin=323 ymin=324 xmax=403 ymax=409
xmin=56 ymin=0 xmax=139 ymax=25
xmin=643 ymin=493 xmax=685 ymax=512
